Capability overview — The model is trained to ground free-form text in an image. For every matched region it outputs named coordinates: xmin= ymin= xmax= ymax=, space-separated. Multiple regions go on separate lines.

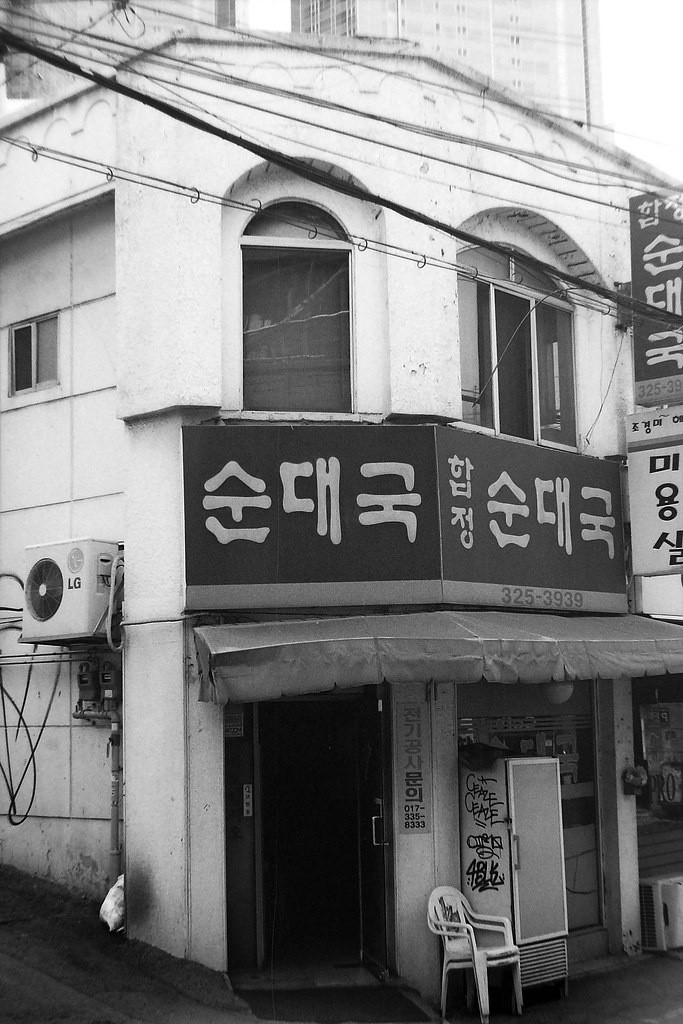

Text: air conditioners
xmin=637 ymin=871 xmax=683 ymax=949
xmin=20 ymin=537 xmax=124 ymax=646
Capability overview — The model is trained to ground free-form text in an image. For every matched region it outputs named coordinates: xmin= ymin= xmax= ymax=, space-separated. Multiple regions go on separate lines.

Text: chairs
xmin=428 ymin=885 xmax=523 ymax=1024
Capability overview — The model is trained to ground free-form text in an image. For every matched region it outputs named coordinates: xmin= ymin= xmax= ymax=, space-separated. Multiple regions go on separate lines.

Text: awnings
xmin=194 ymin=616 xmax=683 ymax=705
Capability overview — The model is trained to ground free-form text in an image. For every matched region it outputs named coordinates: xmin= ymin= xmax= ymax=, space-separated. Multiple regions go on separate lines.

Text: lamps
xmin=540 ymin=681 xmax=573 ymax=704
